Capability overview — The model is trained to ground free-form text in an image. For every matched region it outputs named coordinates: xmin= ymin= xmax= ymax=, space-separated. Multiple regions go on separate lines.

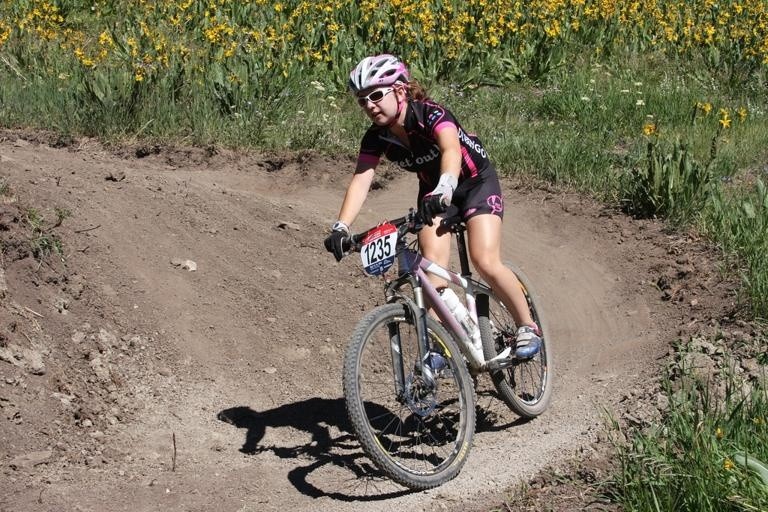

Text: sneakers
xmin=514 ymin=320 xmax=543 ymax=357
xmin=415 ymin=346 xmax=449 ymax=373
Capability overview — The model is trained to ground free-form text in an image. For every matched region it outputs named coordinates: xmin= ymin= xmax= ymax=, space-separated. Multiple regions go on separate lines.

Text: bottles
xmin=439 ymin=285 xmax=482 ymax=343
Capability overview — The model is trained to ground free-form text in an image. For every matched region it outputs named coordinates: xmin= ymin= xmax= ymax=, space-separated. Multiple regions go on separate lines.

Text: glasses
xmin=357 ymin=86 xmax=395 ymax=107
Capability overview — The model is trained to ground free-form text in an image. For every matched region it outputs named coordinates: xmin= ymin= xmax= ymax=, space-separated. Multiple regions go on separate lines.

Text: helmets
xmin=348 ymin=54 xmax=411 ymax=97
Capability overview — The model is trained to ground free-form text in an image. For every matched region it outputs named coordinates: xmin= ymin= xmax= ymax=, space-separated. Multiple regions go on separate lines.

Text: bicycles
xmin=329 ymin=194 xmax=555 ymax=488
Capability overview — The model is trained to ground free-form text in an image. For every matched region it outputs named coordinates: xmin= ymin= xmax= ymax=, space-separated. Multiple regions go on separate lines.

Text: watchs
xmin=332 ymin=221 xmax=349 ymax=233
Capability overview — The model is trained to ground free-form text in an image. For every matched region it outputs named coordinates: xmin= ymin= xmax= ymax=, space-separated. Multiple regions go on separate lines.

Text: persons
xmin=324 ymin=52 xmax=542 ymax=376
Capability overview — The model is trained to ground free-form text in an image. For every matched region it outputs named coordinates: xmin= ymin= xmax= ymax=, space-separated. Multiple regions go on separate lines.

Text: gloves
xmin=324 ymin=220 xmax=351 ymax=261
xmin=418 ymin=173 xmax=460 ymax=226
